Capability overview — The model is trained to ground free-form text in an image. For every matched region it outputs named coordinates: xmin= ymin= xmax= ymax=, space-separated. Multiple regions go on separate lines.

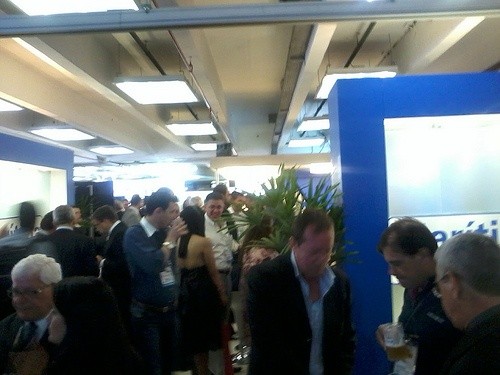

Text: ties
xmin=14 ymin=321 xmax=37 ymax=353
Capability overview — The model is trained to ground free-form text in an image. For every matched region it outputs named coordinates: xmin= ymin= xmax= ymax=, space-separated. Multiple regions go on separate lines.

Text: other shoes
xmin=229 ymin=331 xmax=243 ymax=373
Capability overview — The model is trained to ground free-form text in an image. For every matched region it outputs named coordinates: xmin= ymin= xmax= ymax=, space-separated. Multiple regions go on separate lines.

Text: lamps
xmin=110 ymin=41 xmax=202 ymax=107
xmin=296 ymin=101 xmax=332 ymax=134
xmin=286 ymin=135 xmax=326 ymax=148
xmin=190 ymin=140 xmax=220 ymax=152
xmin=166 ymin=108 xmax=219 ymax=137
xmin=314 ymin=31 xmax=401 ymax=103
xmin=28 ymin=124 xmax=96 ymax=142
xmin=88 ymin=143 xmax=137 ymax=156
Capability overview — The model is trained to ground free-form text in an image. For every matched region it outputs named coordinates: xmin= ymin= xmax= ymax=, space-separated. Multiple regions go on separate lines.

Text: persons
xmin=432 ymin=233 xmax=500 ymax=375
xmin=241 ymin=207 xmax=355 ymax=375
xmin=0 ymin=183 xmax=283 ymax=375
xmin=377 ymin=219 xmax=457 ymax=375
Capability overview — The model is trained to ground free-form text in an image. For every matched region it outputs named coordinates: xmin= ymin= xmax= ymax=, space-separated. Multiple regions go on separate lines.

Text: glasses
xmin=429 ymin=272 xmax=464 ymax=298
xmin=303 ymin=245 xmax=336 ymax=256
xmin=7 ymin=284 xmax=53 ymax=299
xmin=93 ymin=222 xmax=100 ymax=227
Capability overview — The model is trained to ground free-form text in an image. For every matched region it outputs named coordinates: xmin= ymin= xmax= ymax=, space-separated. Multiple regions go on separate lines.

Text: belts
xmin=218 ymin=270 xmax=230 ymax=275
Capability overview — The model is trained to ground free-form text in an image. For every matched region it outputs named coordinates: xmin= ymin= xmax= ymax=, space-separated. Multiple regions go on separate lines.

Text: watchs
xmin=161 ymin=241 xmax=176 ymax=249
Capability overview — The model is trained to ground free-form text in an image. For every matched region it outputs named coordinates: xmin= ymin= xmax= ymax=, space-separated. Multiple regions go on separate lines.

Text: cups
xmin=376 ymin=323 xmax=411 ymax=362
xmin=170 ymin=216 xmax=187 ymax=233
